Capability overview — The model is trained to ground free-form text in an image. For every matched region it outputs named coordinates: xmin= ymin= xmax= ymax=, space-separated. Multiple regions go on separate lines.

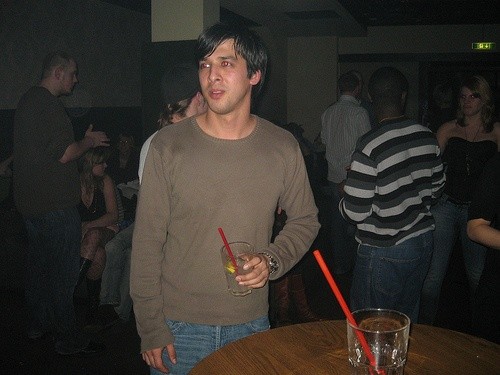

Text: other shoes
xmin=94 ymin=304 xmax=113 ymax=321
xmin=58 ymin=340 xmax=108 ymax=358
xmin=102 ymin=311 xmax=119 ymax=329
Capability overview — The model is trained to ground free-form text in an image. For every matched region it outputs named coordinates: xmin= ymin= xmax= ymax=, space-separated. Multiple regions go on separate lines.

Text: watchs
xmin=259 ymin=251 xmax=279 ymax=277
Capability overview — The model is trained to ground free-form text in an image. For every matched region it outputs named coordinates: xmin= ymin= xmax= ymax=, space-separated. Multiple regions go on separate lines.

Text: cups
xmin=220 ymin=241 xmax=254 ymax=297
xmin=346 ymin=307 xmax=411 ymax=375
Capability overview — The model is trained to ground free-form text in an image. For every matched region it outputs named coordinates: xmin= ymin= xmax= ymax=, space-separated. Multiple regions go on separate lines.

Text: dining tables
xmin=187 ymin=319 xmax=500 ymax=375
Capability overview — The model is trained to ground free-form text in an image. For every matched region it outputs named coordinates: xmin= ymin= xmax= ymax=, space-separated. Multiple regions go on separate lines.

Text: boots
xmin=287 ymin=274 xmax=322 ymax=322
xmin=86 ymin=278 xmax=102 ymax=323
xmin=274 ymin=279 xmax=289 ymax=323
xmin=78 ymin=256 xmax=91 ymax=286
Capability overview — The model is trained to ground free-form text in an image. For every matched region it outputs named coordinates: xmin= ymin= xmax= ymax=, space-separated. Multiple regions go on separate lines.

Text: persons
xmin=418 ymin=77 xmax=500 ymax=325
xmin=14 ymin=41 xmax=111 ymax=358
xmin=320 ymin=73 xmax=372 ymax=209
xmin=350 ymin=70 xmax=375 ymax=130
xmin=138 ymin=63 xmax=208 ymax=184
xmin=285 ymin=122 xmax=313 ymax=184
xmin=421 ymin=66 xmax=458 ymax=133
xmin=77 ymin=134 xmax=146 ymax=328
xmin=329 ymin=67 xmax=447 ymax=325
xmin=451 ymin=151 xmax=500 ymax=346
xmin=129 ymin=22 xmax=321 ymax=375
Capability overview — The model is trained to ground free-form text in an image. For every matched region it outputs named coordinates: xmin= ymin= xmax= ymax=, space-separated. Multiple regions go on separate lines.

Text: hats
xmin=161 ymin=63 xmax=201 ymax=106
